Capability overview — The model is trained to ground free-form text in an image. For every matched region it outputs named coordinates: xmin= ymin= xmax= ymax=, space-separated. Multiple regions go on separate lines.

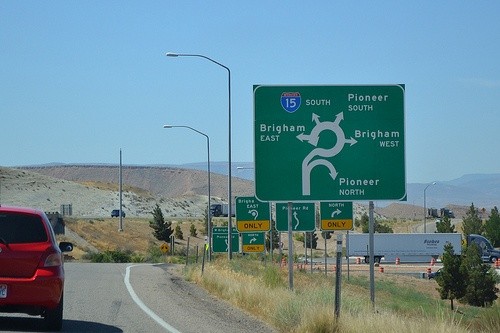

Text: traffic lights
xmin=312 ymin=233 xmax=319 ymax=249
xmin=325 ymin=230 xmax=335 ymax=239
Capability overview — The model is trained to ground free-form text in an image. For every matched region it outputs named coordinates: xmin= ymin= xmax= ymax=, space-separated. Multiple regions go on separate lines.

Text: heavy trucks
xmin=347 ymin=232 xmax=500 ymax=263
xmin=424 ymin=207 xmax=455 ymax=220
xmin=209 ymin=203 xmax=235 ymax=217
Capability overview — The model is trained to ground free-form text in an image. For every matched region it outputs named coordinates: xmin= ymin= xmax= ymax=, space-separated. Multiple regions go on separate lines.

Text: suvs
xmin=0 ymin=206 xmax=73 ymax=330
xmin=111 ymin=209 xmax=126 ymax=218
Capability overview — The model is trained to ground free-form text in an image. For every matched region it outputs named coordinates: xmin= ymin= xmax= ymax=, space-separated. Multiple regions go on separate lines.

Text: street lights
xmin=167 ymin=52 xmax=232 ymax=264
xmin=163 ymin=124 xmax=212 ymax=264
xmin=423 ymin=181 xmax=437 ymax=233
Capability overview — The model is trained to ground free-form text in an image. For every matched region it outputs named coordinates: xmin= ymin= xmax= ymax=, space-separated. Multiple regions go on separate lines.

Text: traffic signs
xmin=276 ymin=202 xmax=316 ymax=233
xmin=235 ymin=195 xmax=272 ymax=232
xmin=212 ymin=227 xmax=240 ymax=253
xmin=241 ymin=231 xmax=266 ymax=254
xmin=320 ymin=201 xmax=353 ymax=231
